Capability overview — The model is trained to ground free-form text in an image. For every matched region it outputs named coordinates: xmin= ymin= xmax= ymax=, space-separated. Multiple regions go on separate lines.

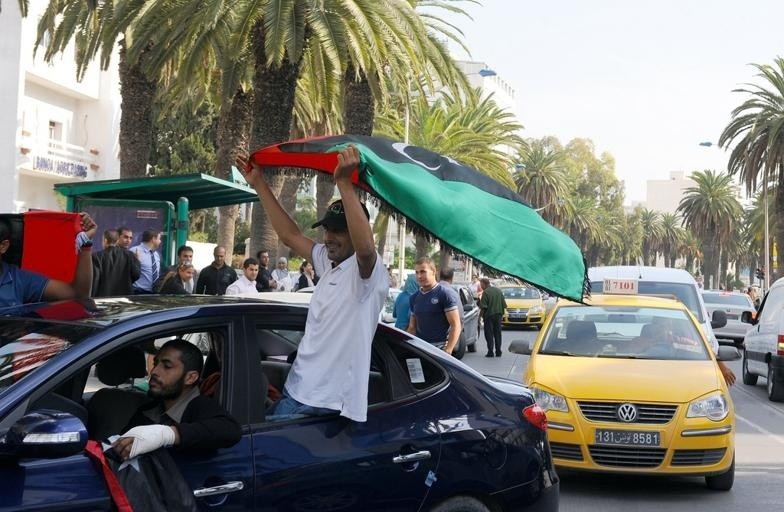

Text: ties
xmin=150 ymin=250 xmax=159 ymax=293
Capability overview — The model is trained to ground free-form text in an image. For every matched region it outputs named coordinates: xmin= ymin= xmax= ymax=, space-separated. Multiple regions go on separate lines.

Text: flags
xmin=243 ymin=134 xmax=592 ymax=308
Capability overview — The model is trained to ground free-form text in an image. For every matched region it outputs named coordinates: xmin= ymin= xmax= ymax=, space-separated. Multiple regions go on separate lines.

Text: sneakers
xmin=485 ymin=350 xmax=502 ymax=357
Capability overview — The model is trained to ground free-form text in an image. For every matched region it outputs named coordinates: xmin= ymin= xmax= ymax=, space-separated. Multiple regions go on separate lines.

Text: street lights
xmin=395 ymin=57 xmax=497 ymax=286
xmin=700 ymin=134 xmax=771 ymax=295
xmin=465 ymin=161 xmax=529 ymax=285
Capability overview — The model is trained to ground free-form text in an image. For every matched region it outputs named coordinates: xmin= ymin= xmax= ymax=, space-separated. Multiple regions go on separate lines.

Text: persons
xmin=622 ymin=318 xmax=736 ymax=388
xmin=233 ymin=145 xmax=390 ymax=424
xmin=1 ymin=212 xmax=508 ymax=462
xmin=747 ymin=287 xmax=755 ymax=301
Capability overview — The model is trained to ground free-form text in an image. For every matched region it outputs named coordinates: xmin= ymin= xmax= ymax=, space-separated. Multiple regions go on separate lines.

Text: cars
xmin=0 ymin=291 xmax=560 ymax=507
xmin=381 ymin=286 xmax=482 ymax=354
xmin=703 ymin=286 xmax=757 ymax=346
xmin=492 ymin=285 xmax=544 ymax=328
xmin=509 ymin=299 xmax=743 ymax=488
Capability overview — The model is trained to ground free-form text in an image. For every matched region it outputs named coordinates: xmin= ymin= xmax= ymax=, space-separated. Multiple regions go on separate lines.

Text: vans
xmin=584 ymin=265 xmax=730 ymax=358
xmin=738 ymin=276 xmax=783 ymax=401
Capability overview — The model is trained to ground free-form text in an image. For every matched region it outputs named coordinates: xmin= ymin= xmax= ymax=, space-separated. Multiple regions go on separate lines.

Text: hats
xmin=312 ymin=199 xmax=369 ymax=231
